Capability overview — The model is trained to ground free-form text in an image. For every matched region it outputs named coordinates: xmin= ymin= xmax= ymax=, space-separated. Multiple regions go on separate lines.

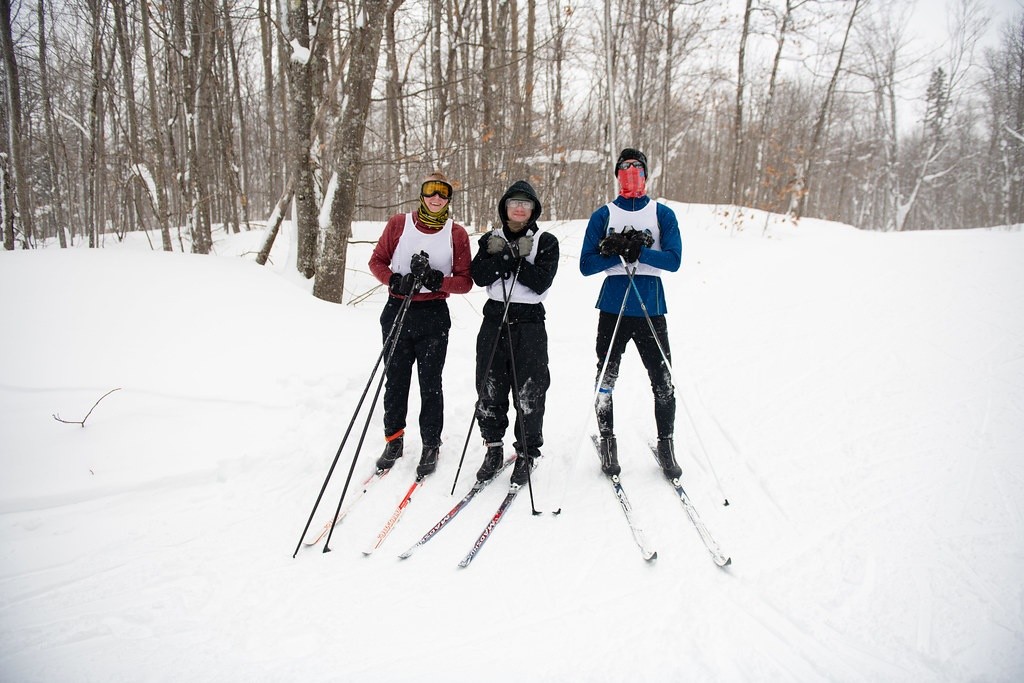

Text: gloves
xmin=600 ymin=232 xmax=626 ymax=257
xmin=486 ymin=235 xmax=517 ymax=271
xmin=409 ymin=254 xmax=444 ymax=293
xmin=623 ymin=232 xmax=655 ymax=264
xmin=388 ymin=272 xmax=420 ymax=297
xmin=509 ymin=236 xmax=534 ymax=258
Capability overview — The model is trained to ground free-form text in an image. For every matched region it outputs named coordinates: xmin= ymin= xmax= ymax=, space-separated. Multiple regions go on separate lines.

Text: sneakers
xmin=659 ymin=437 xmax=682 ymax=481
xmin=601 ymin=437 xmax=621 ymax=475
xmin=476 ymin=445 xmax=503 ymax=482
xmin=375 ymin=436 xmax=404 ymax=469
xmin=417 ymin=444 xmax=440 ymax=475
xmin=510 ymin=454 xmax=535 ymax=486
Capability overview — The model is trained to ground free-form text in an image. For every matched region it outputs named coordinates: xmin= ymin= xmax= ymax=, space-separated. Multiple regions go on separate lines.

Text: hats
xmin=510 ymin=194 xmax=535 ymax=207
xmin=422 ymin=172 xmax=453 ymax=183
xmin=615 ymin=148 xmax=648 ymax=182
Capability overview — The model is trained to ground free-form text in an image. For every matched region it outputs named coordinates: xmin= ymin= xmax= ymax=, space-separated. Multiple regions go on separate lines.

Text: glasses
xmin=506 ymin=199 xmax=534 ymax=209
xmin=619 ymin=161 xmax=644 ymax=170
xmin=420 ymin=180 xmax=452 ymax=199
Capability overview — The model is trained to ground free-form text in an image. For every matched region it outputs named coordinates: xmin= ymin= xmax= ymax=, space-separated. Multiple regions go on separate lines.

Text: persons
xmin=579 ymin=147 xmax=684 ymax=479
xmin=472 ymin=178 xmax=562 ymax=486
xmin=369 ymin=171 xmax=476 ymax=479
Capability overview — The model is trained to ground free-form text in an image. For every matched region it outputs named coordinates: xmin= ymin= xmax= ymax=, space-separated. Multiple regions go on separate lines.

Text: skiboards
xmin=589 ymin=432 xmax=732 ymax=567
xmin=398 ymin=453 xmax=543 ymax=568
xmin=302 ymin=469 xmax=421 ymax=555
